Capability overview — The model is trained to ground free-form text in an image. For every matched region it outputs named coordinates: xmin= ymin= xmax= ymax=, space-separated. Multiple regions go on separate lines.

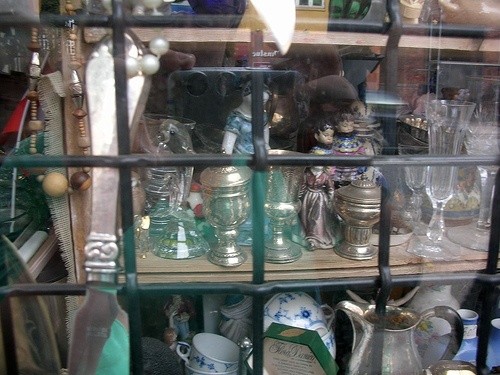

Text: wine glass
xmin=397 ymin=100 xmax=499 ymax=261
xmin=200 ymin=150 xmax=302 ymax=267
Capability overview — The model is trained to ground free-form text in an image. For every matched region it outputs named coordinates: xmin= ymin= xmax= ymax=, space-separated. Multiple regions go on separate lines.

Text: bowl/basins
xmin=176 ymin=292 xmax=336 ymax=375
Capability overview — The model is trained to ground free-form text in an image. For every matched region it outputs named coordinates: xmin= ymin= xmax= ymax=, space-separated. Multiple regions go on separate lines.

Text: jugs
xmin=335 ymin=300 xmax=465 ymax=374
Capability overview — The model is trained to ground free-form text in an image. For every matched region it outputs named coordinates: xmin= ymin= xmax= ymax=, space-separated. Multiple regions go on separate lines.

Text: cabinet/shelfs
xmin=58 ymin=0 xmax=500 ymax=310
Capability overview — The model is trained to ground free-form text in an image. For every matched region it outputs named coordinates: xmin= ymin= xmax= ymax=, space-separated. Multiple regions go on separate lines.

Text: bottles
xmin=409 ymin=283 xmax=500 ymax=374
xmin=0 ymin=26 xmax=62 ymax=74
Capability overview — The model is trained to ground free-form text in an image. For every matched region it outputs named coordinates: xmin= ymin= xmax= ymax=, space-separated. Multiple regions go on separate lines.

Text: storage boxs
xmin=244 ymin=322 xmax=340 ymax=375
